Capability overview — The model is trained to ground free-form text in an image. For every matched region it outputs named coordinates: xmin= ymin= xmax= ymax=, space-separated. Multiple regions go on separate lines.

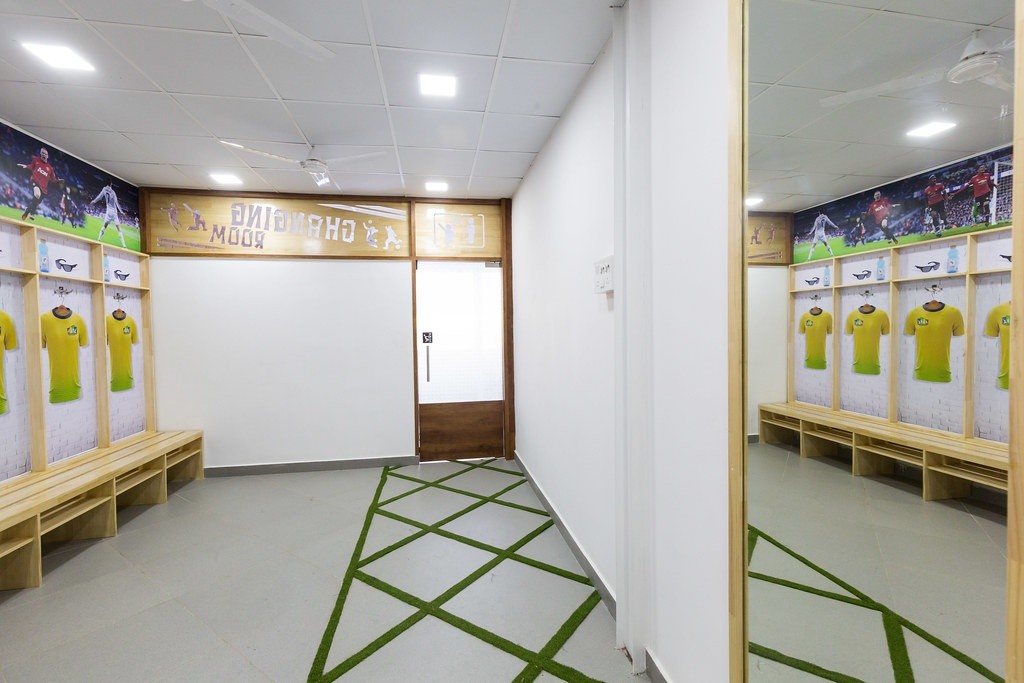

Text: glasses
xmin=852 ymin=270 xmax=871 ymax=280
xmin=114 ymin=270 xmax=130 ymax=281
xmin=805 ymin=277 xmax=819 ymax=286
xmin=1000 ymin=255 xmax=1012 ymax=262
xmin=915 ymin=262 xmax=940 ymax=272
xmin=56 ymin=259 xmax=77 ymax=272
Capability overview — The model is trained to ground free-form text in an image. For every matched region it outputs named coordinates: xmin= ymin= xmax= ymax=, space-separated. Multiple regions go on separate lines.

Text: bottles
xmin=877 ymin=256 xmax=885 ymax=280
xmin=947 ymin=244 xmax=959 ymax=272
xmin=38 ymin=238 xmax=50 ymax=272
xmin=104 ymin=253 xmax=111 ymax=282
xmin=823 ymin=265 xmax=830 ymax=286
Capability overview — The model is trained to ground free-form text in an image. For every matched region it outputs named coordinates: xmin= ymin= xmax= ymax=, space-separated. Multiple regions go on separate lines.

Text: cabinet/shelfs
xmin=1 ymin=217 xmax=157 ymax=477
xmin=787 ymin=225 xmax=1012 ymax=450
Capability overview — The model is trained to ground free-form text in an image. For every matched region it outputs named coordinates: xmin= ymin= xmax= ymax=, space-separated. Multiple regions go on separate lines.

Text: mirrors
xmin=726 ymin=0 xmax=1024 ymax=683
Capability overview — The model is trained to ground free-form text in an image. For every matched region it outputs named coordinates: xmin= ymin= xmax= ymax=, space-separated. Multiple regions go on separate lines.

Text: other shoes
xmin=894 ymin=238 xmax=898 ymax=244
xmin=22 ymin=211 xmax=27 ymax=220
xmin=30 ymin=213 xmax=34 ymax=220
xmin=888 ymin=239 xmax=892 ymax=243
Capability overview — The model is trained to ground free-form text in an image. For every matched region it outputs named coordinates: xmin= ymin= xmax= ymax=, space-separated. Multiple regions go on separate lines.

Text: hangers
xmin=861 ymin=293 xmax=872 ymax=311
xmin=811 ymin=300 xmax=820 ymax=313
xmin=926 ymin=292 xmax=941 ymax=308
xmin=115 ymin=301 xmax=124 ymax=315
xmin=55 ymin=296 xmax=69 ymax=314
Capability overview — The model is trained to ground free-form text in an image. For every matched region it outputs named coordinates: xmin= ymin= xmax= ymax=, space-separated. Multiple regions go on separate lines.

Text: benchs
xmin=0 ymin=430 xmax=204 ymax=592
xmin=756 ymin=402 xmax=1011 ymax=502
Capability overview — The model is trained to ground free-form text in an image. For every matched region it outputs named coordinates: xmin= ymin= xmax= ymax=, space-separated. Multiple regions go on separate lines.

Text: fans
xmin=819 ymin=28 xmax=1016 ymax=108
xmin=184 ymin=0 xmax=338 ymax=61
xmin=220 ymin=139 xmax=387 ymax=191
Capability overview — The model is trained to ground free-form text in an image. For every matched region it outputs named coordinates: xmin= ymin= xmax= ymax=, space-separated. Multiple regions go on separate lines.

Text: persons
xmin=60 ymin=186 xmax=78 ymax=226
xmin=924 ymin=174 xmax=949 ymax=238
xmin=867 ymin=191 xmax=901 ymax=244
xmin=90 ymin=178 xmax=128 ymax=248
xmin=17 ymin=147 xmax=64 ymax=220
xmin=807 ymin=210 xmax=839 ymax=260
xmin=950 ymin=164 xmax=999 ymax=227
xmin=850 ymin=217 xmax=866 ymax=246
